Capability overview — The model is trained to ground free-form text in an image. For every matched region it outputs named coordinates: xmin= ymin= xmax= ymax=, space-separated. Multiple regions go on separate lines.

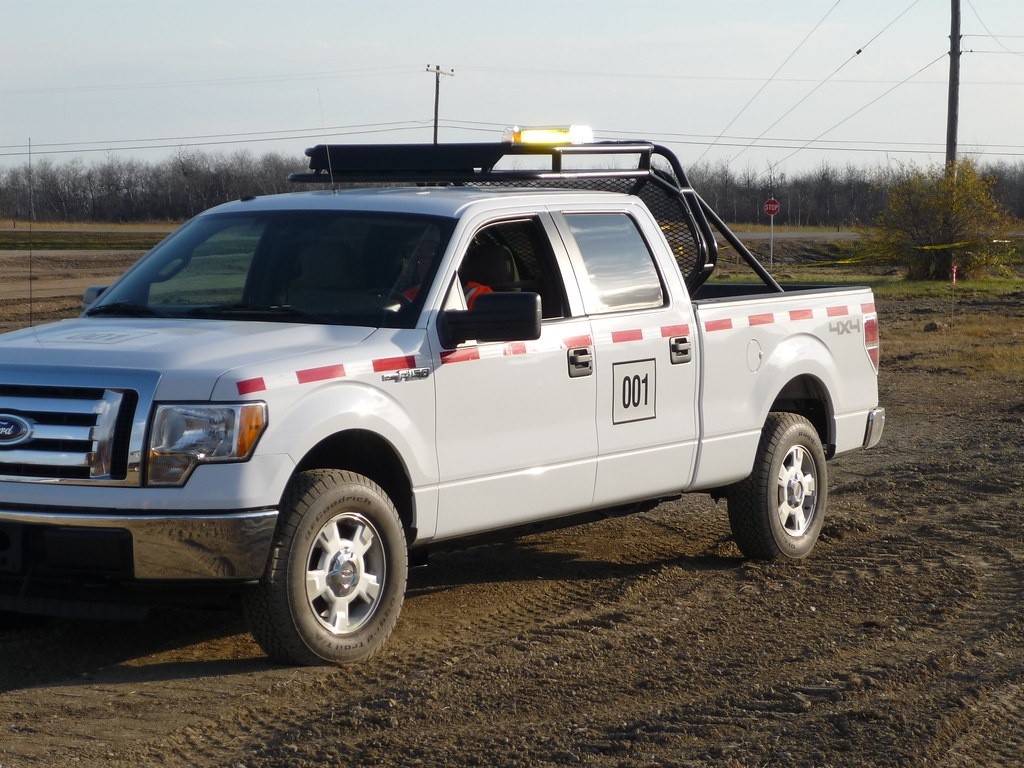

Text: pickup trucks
xmin=0 ymin=126 xmax=887 ymax=671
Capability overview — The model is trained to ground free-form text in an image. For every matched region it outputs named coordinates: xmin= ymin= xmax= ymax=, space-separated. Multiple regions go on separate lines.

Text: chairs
xmin=458 ymin=240 xmax=522 ymax=294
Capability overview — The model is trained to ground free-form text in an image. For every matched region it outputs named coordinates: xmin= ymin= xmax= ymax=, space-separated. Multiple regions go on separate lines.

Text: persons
xmin=390 ymin=253 xmax=494 ymax=314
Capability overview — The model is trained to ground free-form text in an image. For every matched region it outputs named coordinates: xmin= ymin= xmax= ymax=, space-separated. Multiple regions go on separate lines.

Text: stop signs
xmin=764 ymin=199 xmax=780 ymax=216
